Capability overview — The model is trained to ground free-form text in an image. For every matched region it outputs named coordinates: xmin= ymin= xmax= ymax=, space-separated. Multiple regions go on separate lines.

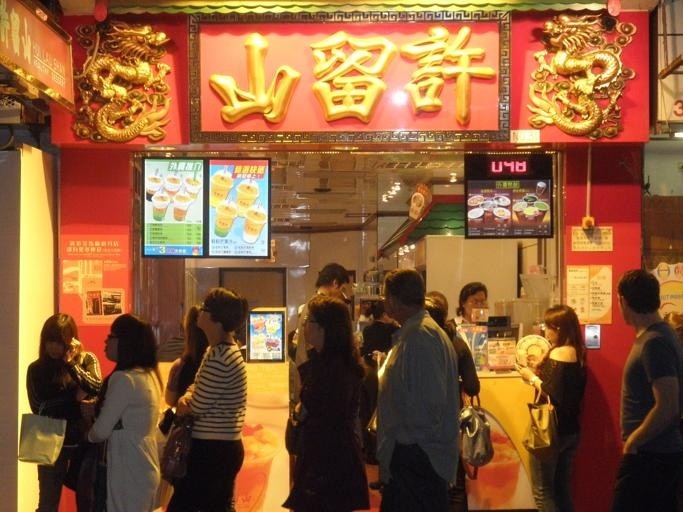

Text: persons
xmin=513 ymin=303 xmax=586 ymax=511
xmin=163 ymin=302 xmax=209 ymax=408
xmin=87 ymin=312 xmax=162 ymax=512
xmin=285 ymin=261 xmax=495 ymax=494
xmin=159 ymin=285 xmax=248 ymax=512
xmin=606 ymin=269 xmax=682 ymax=511
xmin=279 ymin=295 xmax=371 ymax=511
xmin=371 ymin=267 xmax=460 ymax=511
xmin=23 ymin=311 xmax=103 ymax=512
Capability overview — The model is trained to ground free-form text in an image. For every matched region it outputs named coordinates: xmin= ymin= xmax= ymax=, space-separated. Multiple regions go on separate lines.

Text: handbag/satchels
xmin=461 ymin=405 xmax=494 ymax=467
xmin=18 ymin=413 xmax=68 ymax=468
xmin=522 ymin=402 xmax=562 ymax=459
xmin=163 ymin=419 xmax=191 ymax=481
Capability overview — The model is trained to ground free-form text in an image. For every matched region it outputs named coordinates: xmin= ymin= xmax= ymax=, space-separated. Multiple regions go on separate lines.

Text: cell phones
xmin=515 ymin=362 xmax=527 ymax=369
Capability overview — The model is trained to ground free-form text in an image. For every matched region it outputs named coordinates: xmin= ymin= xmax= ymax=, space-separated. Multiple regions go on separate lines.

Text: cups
xmin=466 ymin=180 xmax=550 ymax=231
xmin=145 ymin=168 xmax=268 ymax=246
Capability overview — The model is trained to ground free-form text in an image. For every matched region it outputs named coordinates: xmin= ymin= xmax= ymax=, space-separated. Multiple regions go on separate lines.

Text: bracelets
xmin=529 ymin=376 xmax=539 ymax=386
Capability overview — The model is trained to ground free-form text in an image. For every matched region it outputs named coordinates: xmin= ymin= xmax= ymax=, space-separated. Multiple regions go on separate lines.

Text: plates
xmin=516 ymin=333 xmax=553 ymax=368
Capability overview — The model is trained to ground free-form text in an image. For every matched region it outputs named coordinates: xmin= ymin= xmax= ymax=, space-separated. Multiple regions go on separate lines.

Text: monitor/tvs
xmin=479 ymin=324 xmax=519 ymax=343
